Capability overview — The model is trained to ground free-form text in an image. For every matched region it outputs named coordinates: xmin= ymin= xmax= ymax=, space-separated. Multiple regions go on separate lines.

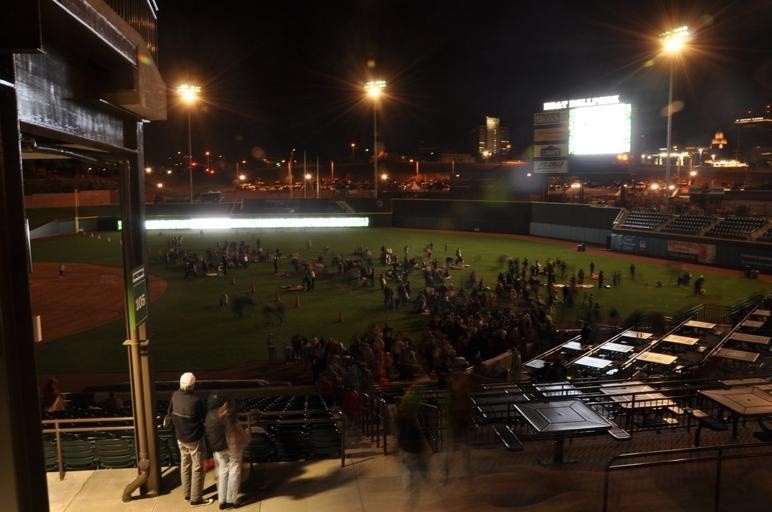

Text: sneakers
xmin=184 ymin=497 xmax=240 ymax=509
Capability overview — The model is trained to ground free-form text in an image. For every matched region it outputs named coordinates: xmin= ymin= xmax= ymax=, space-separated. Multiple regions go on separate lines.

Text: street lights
xmin=178 ymin=84 xmax=202 ymax=204
xmin=364 ymin=80 xmax=387 ymax=199
xmin=658 ymin=25 xmax=690 ymax=179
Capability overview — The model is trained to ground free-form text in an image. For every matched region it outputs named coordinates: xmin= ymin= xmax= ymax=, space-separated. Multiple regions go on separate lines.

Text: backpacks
xmin=162 ymin=416 xmax=172 ymax=430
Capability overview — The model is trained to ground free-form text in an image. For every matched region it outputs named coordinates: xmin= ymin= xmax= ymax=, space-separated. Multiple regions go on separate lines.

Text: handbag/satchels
xmin=225 ymin=424 xmax=249 ymax=453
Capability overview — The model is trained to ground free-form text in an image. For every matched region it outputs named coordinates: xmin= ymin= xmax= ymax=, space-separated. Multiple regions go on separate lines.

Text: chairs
xmin=42 ymin=395 xmax=340 ymax=473
xmin=621 ymin=207 xmax=772 ymax=242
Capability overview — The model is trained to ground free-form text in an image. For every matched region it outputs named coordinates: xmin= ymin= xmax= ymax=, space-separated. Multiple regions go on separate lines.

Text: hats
xmin=208 ymin=394 xmax=225 ymax=409
xmin=180 ymin=372 xmax=196 ymax=385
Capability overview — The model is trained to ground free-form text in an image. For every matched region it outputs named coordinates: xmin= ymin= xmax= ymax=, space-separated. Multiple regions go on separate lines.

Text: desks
xmin=470 ymin=308 xmax=772 ymax=467
